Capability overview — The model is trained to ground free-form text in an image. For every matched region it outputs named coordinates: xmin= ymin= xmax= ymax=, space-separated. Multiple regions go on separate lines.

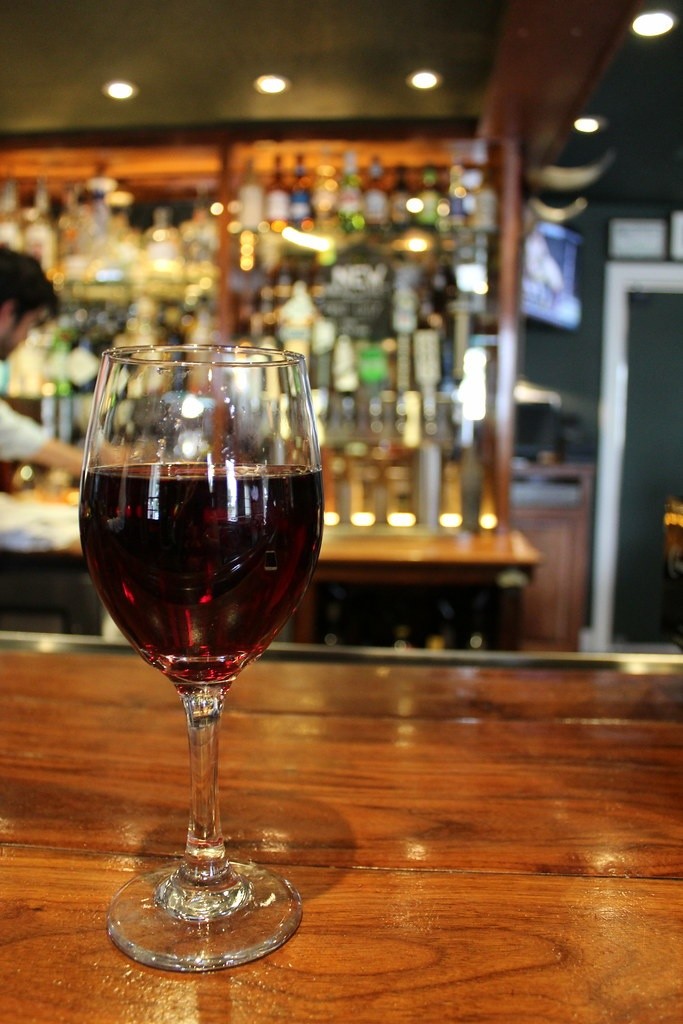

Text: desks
xmin=0 ymin=629 xmax=683 ymax=1024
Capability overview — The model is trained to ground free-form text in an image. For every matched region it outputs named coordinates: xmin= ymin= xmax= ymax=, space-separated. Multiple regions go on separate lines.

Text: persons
xmin=0 ymin=245 xmax=131 ymax=485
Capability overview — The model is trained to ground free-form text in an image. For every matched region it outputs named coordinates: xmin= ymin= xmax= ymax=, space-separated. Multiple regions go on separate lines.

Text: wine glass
xmin=78 ymin=342 xmax=326 ymax=973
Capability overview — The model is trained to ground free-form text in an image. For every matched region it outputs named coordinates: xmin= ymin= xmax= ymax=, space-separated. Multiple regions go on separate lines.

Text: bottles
xmin=238 ymin=152 xmax=482 ymax=228
